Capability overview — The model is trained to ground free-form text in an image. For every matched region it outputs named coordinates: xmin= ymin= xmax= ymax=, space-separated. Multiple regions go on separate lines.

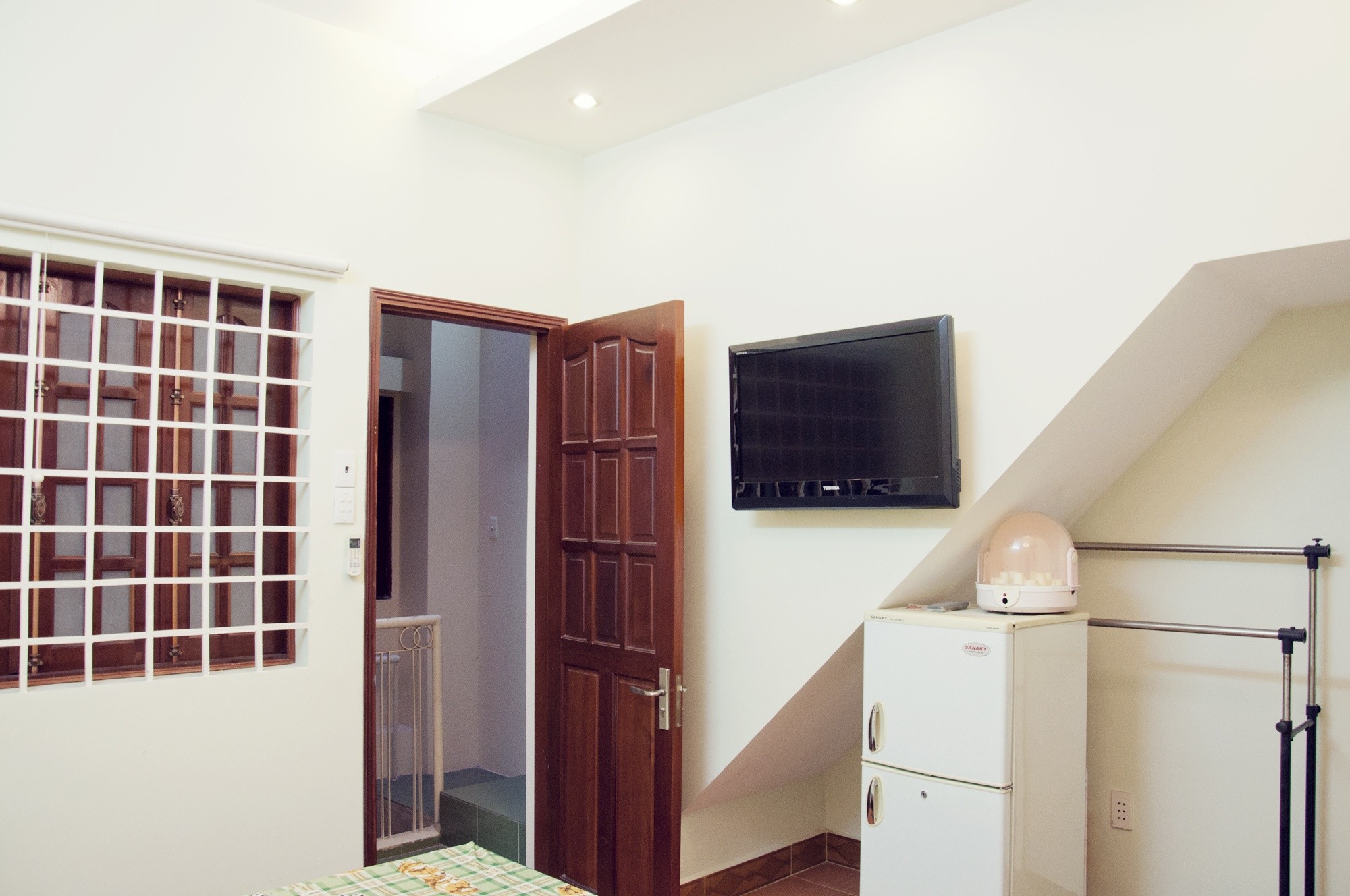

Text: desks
xmin=248 ymin=841 xmax=596 ymax=896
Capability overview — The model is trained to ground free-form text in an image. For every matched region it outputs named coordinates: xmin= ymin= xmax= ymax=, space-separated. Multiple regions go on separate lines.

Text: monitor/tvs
xmin=728 ymin=315 xmax=960 ymax=510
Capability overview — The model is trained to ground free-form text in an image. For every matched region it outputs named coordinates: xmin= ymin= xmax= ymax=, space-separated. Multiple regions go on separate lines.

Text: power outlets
xmin=1110 ymin=791 xmax=1133 ymax=829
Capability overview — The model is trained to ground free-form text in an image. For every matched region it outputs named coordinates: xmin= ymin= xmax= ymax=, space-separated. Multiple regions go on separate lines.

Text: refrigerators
xmin=859 ymin=602 xmax=1092 ymax=896
